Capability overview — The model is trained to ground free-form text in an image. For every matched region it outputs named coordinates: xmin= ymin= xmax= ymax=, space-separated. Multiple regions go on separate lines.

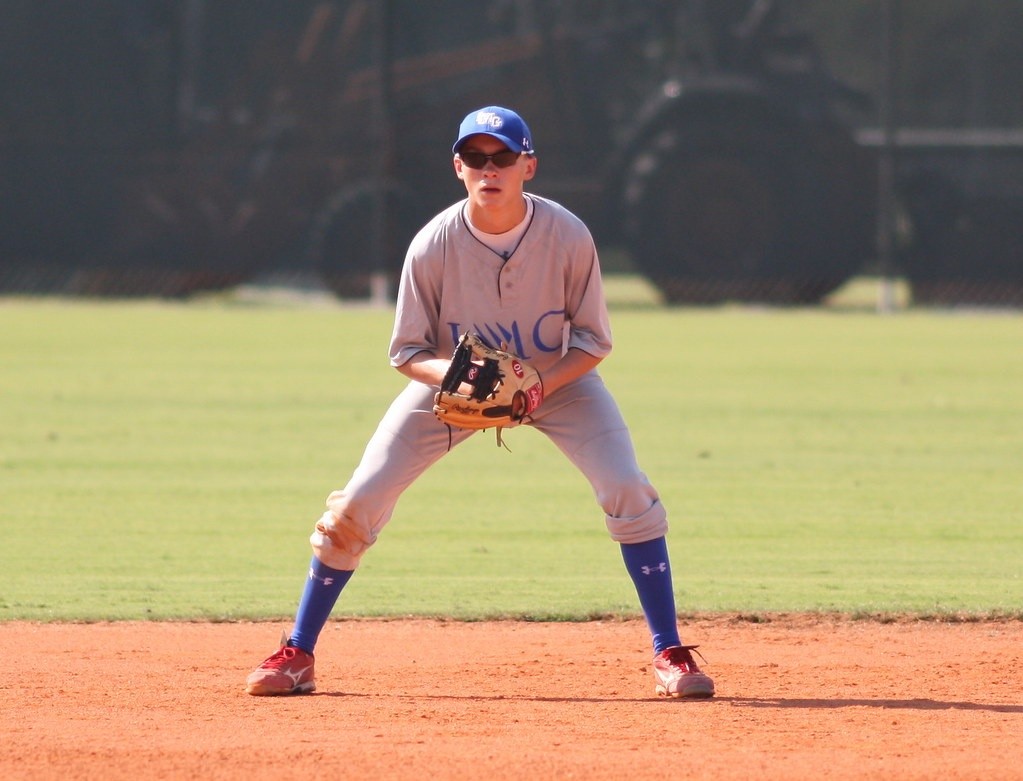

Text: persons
xmin=246 ymin=106 xmax=716 ymax=696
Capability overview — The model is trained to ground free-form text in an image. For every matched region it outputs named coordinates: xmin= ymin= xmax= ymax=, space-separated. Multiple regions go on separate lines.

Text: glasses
xmin=459 ymin=150 xmax=535 ymax=170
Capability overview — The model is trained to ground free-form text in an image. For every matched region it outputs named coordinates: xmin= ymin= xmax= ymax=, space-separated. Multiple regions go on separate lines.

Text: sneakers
xmin=652 ymin=645 xmax=715 ymax=699
xmin=247 ymin=630 xmax=316 ymax=695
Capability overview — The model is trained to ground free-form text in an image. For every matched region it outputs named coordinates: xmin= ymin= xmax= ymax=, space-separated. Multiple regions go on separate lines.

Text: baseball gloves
xmin=433 ymin=333 xmax=543 ymax=429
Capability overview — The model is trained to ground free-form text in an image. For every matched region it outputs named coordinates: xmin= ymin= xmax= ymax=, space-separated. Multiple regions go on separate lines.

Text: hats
xmin=451 ymin=106 xmax=532 ymax=153
